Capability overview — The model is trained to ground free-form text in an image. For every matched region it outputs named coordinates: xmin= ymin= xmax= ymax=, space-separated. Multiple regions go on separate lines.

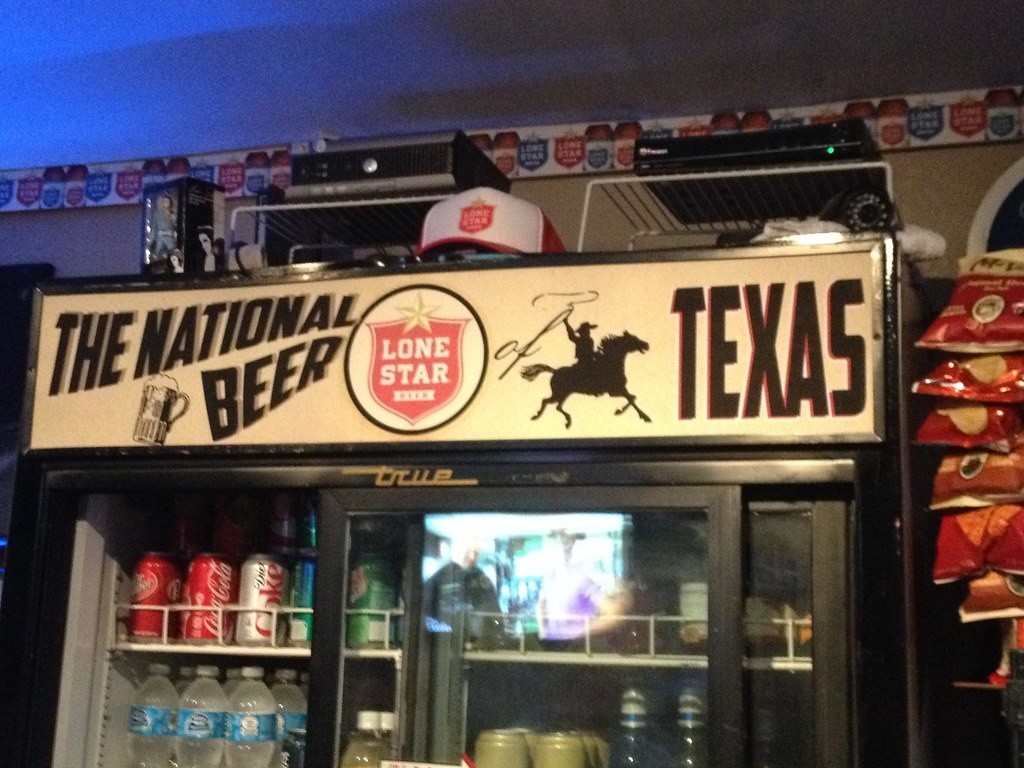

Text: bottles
xmin=668 ymin=674 xmax=710 ymax=768
xmin=611 ymin=673 xmax=652 ymax=768
xmin=124 ymin=666 xmax=397 ymax=768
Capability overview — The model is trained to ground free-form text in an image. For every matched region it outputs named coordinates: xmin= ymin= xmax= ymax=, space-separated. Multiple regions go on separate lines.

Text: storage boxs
xmin=140 ymin=175 xmax=226 ymax=280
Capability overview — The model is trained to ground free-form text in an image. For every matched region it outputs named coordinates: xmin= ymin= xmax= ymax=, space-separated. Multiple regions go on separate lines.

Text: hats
xmin=412 ymin=186 xmax=566 ymax=255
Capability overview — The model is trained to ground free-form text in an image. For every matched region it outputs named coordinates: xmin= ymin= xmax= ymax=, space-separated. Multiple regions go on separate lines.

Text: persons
xmin=425 ymin=526 xmax=622 ymax=649
xmin=194 ymin=225 xmax=215 ymax=272
xmin=147 ymin=195 xmax=176 ymax=258
xmin=169 ymin=249 xmax=183 ymax=272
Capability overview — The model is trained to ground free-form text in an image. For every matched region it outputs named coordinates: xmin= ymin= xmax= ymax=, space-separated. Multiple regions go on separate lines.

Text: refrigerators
xmin=48 ymin=460 xmax=861 ymax=768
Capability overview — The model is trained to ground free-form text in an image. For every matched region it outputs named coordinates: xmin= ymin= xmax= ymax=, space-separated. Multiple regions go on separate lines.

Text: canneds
xmin=471 ymin=724 xmax=618 ymax=768
xmin=128 ymin=486 xmax=400 ymax=648
